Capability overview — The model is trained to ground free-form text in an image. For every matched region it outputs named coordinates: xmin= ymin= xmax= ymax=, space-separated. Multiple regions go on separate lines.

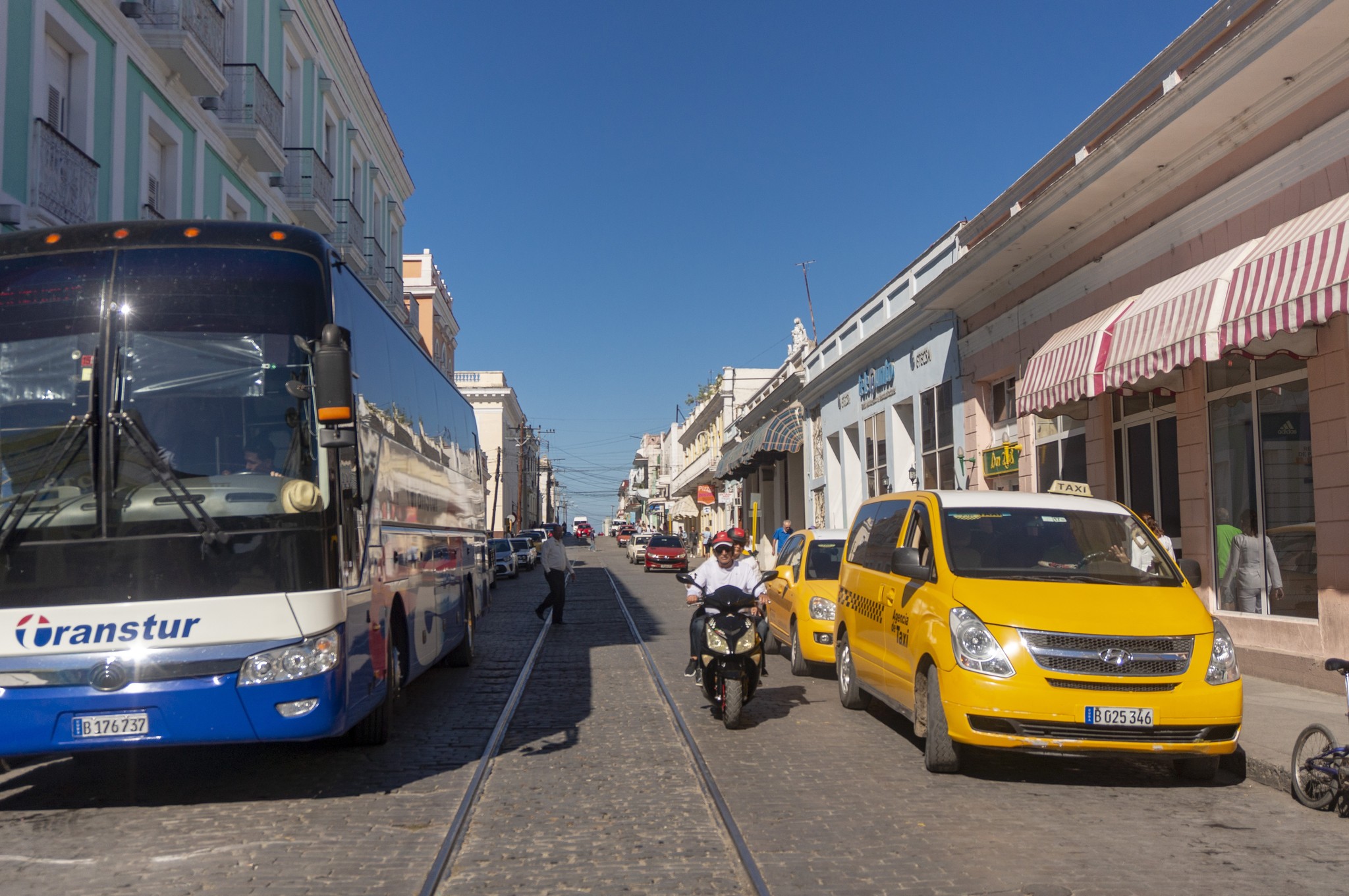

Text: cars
xmin=576 ymin=524 xmax=593 ymax=538
xmin=761 ymin=528 xmax=851 ymax=675
xmin=616 ymin=525 xmax=639 ymax=548
xmin=486 ymin=524 xmax=562 ymax=578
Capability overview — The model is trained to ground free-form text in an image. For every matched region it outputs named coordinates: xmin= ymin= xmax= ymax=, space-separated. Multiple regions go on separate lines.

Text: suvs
xmin=626 ymin=532 xmax=664 ymax=564
xmin=642 ymin=533 xmax=690 ymax=572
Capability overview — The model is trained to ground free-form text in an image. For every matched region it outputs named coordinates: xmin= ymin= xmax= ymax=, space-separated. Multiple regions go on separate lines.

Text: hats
xmin=713 ymin=541 xmax=733 ymax=548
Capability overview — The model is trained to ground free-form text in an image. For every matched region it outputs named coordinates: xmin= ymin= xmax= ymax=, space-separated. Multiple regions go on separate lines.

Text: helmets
xmin=727 ymin=528 xmax=747 ymax=542
xmin=713 ymin=532 xmax=734 ymax=543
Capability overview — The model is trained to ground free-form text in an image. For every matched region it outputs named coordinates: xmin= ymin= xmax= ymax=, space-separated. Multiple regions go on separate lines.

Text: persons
xmin=598 ymin=532 xmax=604 ymax=536
xmin=677 ymin=525 xmax=713 ymax=559
xmin=504 ymin=531 xmax=514 ymax=538
xmin=632 ymin=520 xmax=667 ymax=533
xmin=221 ymin=439 xmax=284 ymax=476
xmin=684 ymin=527 xmax=769 ymax=676
xmin=535 ymin=524 xmax=576 ymax=625
xmin=743 ymin=528 xmax=752 ymax=552
xmin=562 ymin=521 xmax=566 ymax=534
xmin=588 ymin=530 xmax=597 ymax=552
xmin=1216 ymin=508 xmax=1284 ymax=613
xmin=683 ymin=532 xmax=769 ymax=688
xmin=772 ymin=520 xmax=794 ymax=556
xmin=1131 ymin=512 xmax=1176 ymax=575
xmin=532 ymin=521 xmax=544 ymax=528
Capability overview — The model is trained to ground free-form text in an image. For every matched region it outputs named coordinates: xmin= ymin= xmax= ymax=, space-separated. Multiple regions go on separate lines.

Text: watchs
xmin=761 ymin=592 xmax=766 ymax=594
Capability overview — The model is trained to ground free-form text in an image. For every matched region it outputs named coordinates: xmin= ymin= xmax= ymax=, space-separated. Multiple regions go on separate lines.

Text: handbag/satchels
xmin=707 ymin=537 xmax=712 ymax=546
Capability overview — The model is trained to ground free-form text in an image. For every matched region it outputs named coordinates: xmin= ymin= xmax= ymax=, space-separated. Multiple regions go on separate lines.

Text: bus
xmin=0 ymin=222 xmax=486 ymax=761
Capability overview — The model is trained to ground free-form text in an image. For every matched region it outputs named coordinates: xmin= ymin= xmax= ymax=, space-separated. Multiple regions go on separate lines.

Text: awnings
xmin=1018 ymin=193 xmax=1349 ymax=421
xmin=713 ymin=406 xmax=804 ymax=481
xmin=667 ymin=493 xmax=700 ymax=521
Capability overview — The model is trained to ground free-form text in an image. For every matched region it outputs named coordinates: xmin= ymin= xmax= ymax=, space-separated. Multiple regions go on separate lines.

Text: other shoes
xmin=588 ymin=548 xmax=590 ymax=551
xmin=535 ymin=609 xmax=545 ymax=621
xmin=758 ymin=681 xmax=763 ymax=686
xmin=693 ymin=555 xmax=695 ymax=558
xmin=552 ymin=621 xmax=566 ymax=626
xmin=696 ymin=669 xmax=703 ymax=686
xmin=593 ymin=550 xmax=596 ymax=552
xmin=690 ymin=554 xmax=693 ymax=558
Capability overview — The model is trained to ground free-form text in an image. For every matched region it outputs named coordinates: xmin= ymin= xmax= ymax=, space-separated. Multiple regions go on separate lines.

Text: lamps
xmin=200 ymin=97 xmax=218 ymax=110
xmin=1002 ymin=432 xmax=1022 ymax=467
xmin=269 ymin=177 xmax=285 ymax=187
xmin=909 ymin=464 xmax=919 ymax=486
xmin=119 ymin=2 xmax=142 ymax=19
xmin=881 ymin=473 xmax=892 ymax=491
xmin=957 ymin=447 xmax=975 ymax=476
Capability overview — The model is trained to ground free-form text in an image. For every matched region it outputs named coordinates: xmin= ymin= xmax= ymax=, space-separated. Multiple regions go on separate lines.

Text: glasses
xmin=715 ymin=546 xmax=731 ymax=553
xmin=733 ymin=540 xmax=745 ymax=547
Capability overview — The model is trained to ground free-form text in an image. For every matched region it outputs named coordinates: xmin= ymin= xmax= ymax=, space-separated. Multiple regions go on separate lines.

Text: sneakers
xmin=761 ymin=669 xmax=769 ymax=677
xmin=684 ymin=658 xmax=698 ymax=677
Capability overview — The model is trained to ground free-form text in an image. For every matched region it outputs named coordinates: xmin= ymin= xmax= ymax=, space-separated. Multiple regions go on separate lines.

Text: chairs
xmin=946 ymin=517 xmax=982 ymax=569
xmin=1035 ymin=514 xmax=1085 ymax=564
xmin=267 ymin=431 xmax=294 ymax=468
xmin=812 ymin=552 xmax=835 ymax=578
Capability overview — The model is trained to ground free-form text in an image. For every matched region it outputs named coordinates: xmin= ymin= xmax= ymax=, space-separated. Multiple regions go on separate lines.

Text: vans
xmin=833 ymin=481 xmax=1244 ymax=775
xmin=611 ymin=518 xmax=626 ymax=536
xmin=571 ymin=516 xmax=588 ymax=536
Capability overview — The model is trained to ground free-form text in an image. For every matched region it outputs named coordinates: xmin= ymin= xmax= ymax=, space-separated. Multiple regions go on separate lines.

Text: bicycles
xmin=1291 ymin=658 xmax=1349 ymax=816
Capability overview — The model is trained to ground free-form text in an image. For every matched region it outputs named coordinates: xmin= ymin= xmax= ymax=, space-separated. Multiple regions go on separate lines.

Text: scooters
xmin=675 ymin=571 xmax=779 ymax=728
